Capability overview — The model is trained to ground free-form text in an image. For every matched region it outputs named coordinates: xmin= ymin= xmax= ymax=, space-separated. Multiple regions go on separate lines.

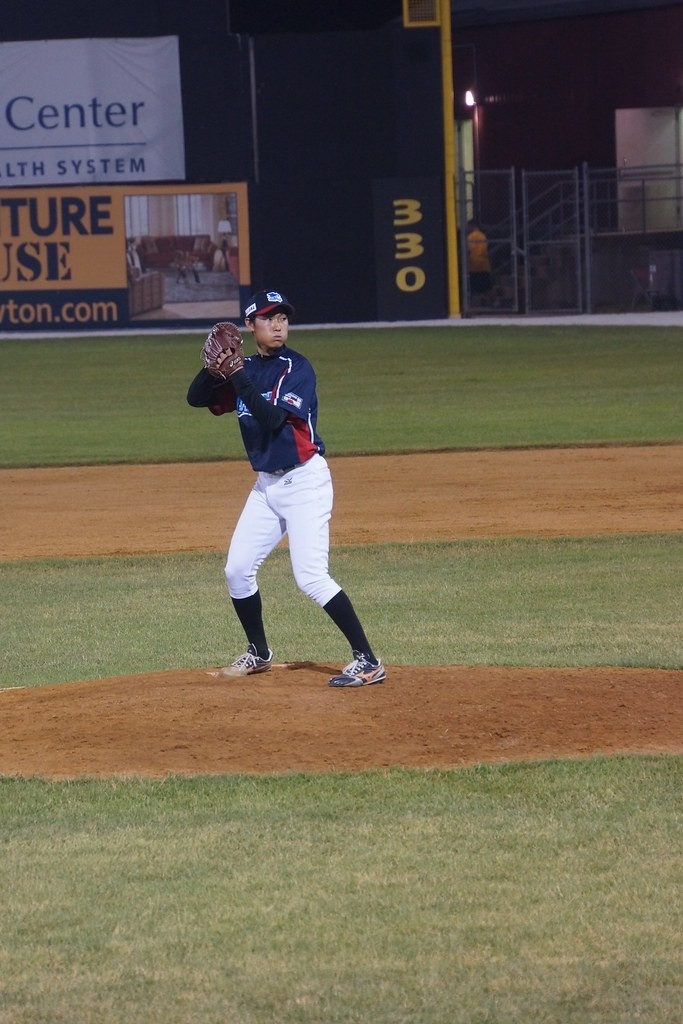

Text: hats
xmin=244 ymin=288 xmax=295 ymax=321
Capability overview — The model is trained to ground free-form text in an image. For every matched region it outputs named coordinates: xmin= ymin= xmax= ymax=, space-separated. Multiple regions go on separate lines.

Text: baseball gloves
xmin=201 ymin=321 xmax=245 ymax=377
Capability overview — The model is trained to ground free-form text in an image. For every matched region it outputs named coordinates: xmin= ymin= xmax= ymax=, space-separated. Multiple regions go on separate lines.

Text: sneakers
xmin=328 ymin=650 xmax=386 ymax=688
xmin=222 ymin=643 xmax=274 ymax=676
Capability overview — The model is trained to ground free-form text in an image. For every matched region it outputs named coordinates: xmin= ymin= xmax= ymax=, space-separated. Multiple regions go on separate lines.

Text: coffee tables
xmin=171 ymin=263 xmax=205 ymax=284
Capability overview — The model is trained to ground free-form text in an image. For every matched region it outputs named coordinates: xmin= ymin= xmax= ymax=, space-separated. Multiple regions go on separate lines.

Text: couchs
xmin=126 ymin=256 xmax=164 ymax=316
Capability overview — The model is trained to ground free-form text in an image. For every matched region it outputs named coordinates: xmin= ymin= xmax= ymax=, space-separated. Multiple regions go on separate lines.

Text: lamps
xmin=219 ymin=219 xmax=232 ymax=254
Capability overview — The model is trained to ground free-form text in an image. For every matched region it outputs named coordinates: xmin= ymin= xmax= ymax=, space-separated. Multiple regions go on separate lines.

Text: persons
xmin=187 ymin=291 xmax=388 ymax=687
xmin=464 ymin=218 xmax=493 ymax=293
